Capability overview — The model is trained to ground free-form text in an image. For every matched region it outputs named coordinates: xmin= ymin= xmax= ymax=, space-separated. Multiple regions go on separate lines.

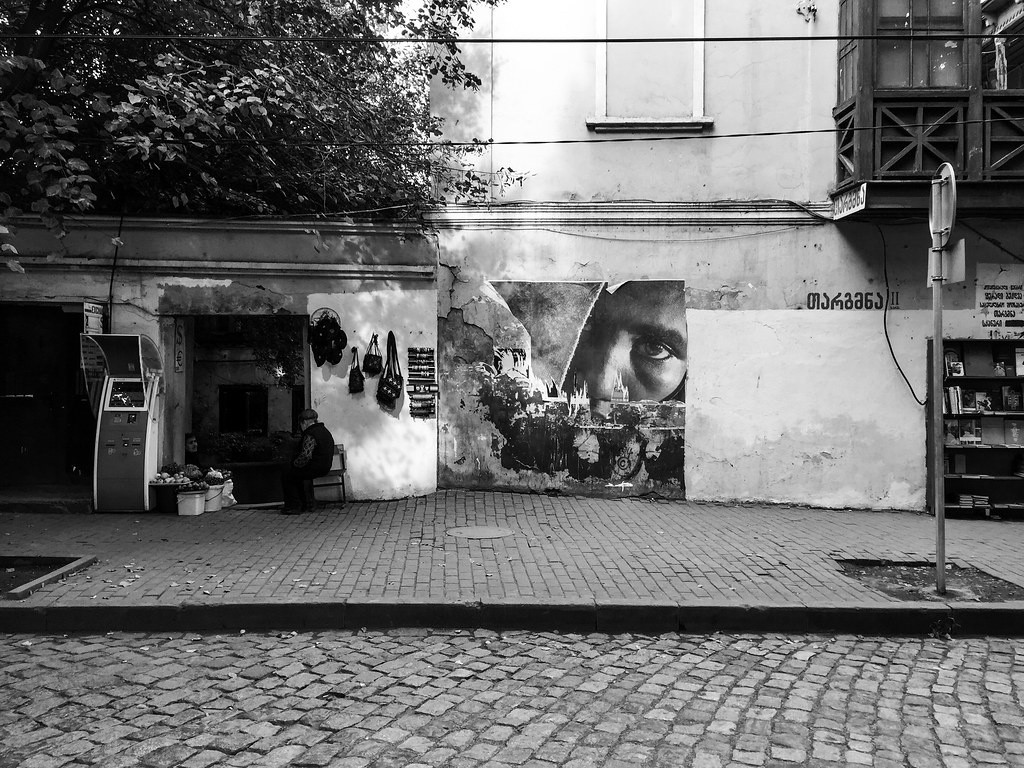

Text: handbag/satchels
xmin=376 ymin=332 xmax=403 ymax=409
xmin=349 ymin=348 xmax=364 ymax=393
xmin=362 ymin=334 xmax=382 ymax=375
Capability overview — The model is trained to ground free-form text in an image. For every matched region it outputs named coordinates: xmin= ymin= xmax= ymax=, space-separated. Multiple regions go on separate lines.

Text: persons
xmin=185 ymin=432 xmax=197 ymax=464
xmin=281 ymin=408 xmax=334 ymax=514
xmin=561 ymin=280 xmax=686 ymax=421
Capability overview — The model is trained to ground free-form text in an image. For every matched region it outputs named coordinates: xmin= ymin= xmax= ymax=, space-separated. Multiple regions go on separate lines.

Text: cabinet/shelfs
xmin=941 ymin=374 xmax=1024 ymax=516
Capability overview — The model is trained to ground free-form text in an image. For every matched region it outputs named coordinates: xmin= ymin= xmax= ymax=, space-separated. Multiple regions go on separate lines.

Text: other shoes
xmin=281 ymin=508 xmax=300 ymax=515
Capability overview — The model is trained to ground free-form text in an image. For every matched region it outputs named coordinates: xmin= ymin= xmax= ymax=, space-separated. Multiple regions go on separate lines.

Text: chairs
xmin=310 ymin=444 xmax=349 ymax=510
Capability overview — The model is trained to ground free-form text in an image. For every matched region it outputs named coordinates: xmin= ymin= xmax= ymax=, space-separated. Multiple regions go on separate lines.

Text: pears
xmin=150 ymin=473 xmax=189 ymax=483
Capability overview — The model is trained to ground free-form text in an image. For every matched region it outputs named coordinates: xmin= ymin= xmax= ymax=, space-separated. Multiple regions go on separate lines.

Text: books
xmin=943 ymin=346 xmax=1024 ymax=509
